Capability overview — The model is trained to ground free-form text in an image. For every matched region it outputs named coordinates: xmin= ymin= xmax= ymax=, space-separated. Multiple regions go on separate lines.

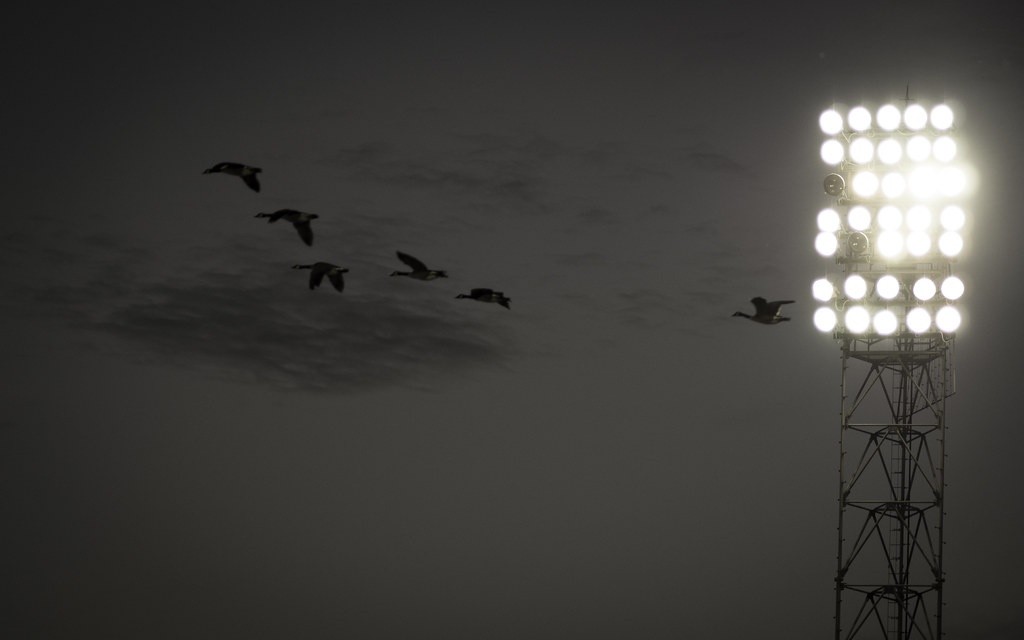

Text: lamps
xmin=823 ymin=172 xmax=845 ymax=197
xmin=847 ymin=232 xmax=869 ymax=253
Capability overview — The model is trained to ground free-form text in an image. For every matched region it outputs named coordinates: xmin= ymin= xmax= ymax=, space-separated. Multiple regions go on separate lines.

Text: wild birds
xmin=290 ymin=261 xmax=350 ymax=293
xmin=202 ymin=162 xmax=263 ymax=193
xmin=731 ymin=296 xmax=796 ymax=325
xmin=454 ymin=288 xmax=512 ymax=310
xmin=390 ymin=250 xmax=450 ymax=280
xmin=253 ymin=208 xmax=320 ymax=247
